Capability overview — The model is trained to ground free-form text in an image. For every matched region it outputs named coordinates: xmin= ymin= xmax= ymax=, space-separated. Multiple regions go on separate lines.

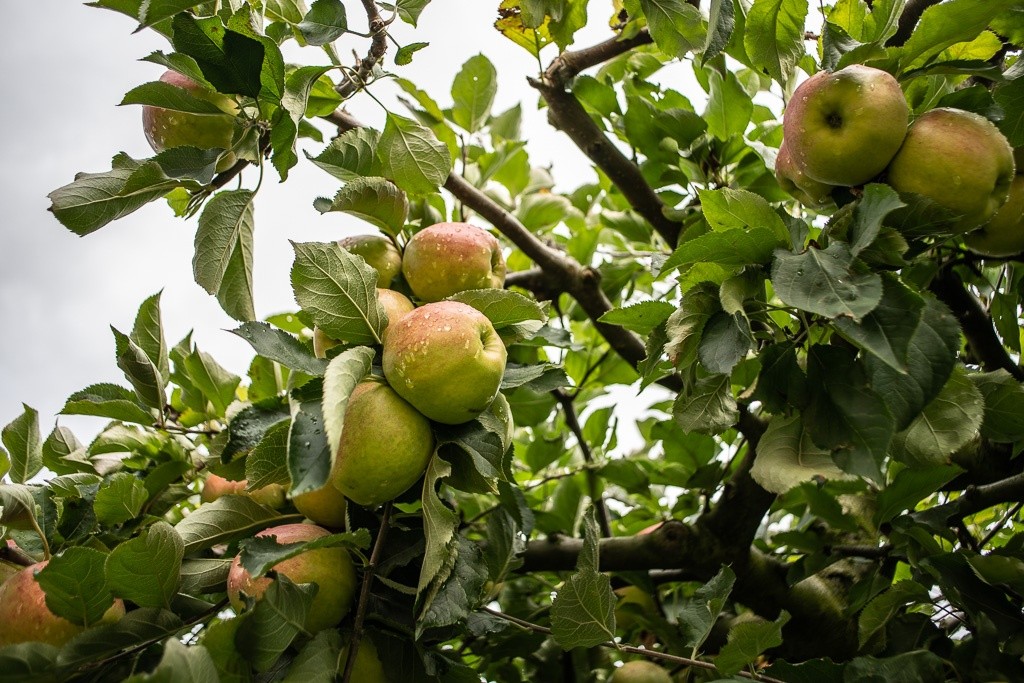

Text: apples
xmin=606 ymin=659 xmax=671 ymax=683
xmin=0 ymin=557 xmax=128 ymax=655
xmin=141 ymin=67 xmax=247 ymax=177
xmin=200 ymin=221 xmax=508 ymax=630
xmin=773 ymin=64 xmax=1024 ymax=261
